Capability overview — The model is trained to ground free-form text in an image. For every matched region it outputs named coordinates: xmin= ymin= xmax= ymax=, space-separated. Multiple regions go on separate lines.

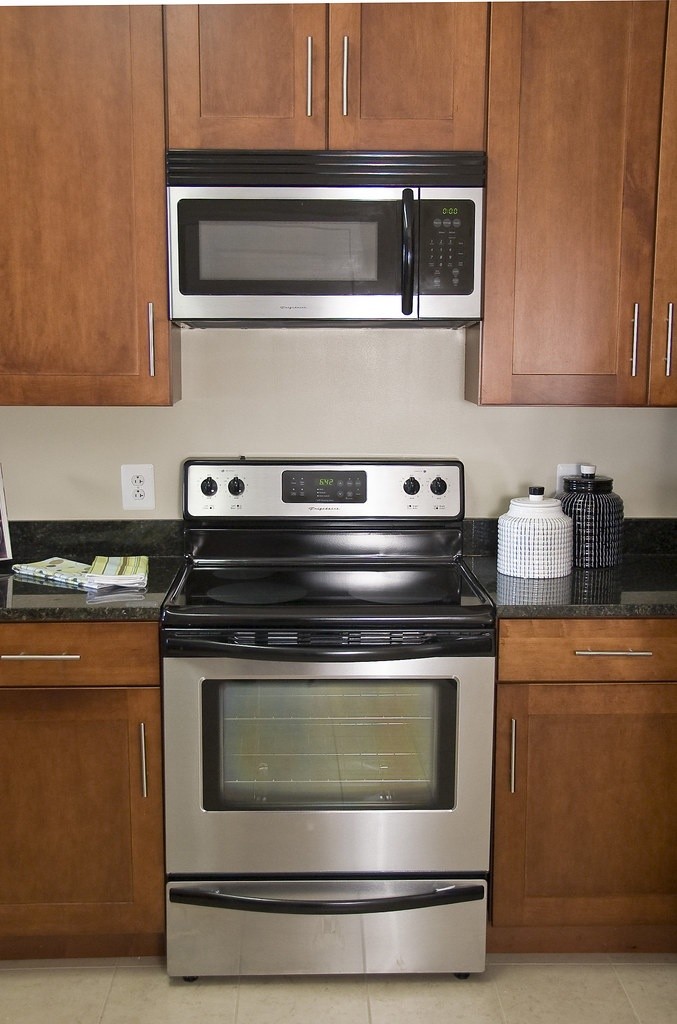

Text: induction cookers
xmin=162 ymin=457 xmax=494 ymax=627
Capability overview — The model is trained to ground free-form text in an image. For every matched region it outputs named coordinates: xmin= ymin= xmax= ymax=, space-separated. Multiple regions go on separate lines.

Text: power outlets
xmin=120 ymin=464 xmax=154 ymax=511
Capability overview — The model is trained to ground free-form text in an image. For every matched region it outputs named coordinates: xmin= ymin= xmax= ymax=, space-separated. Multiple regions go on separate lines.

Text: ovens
xmin=158 ymin=624 xmax=498 ymax=981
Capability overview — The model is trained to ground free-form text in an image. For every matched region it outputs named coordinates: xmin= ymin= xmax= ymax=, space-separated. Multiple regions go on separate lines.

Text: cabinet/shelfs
xmin=162 ymin=3 xmax=491 ymax=153
xmin=0 ymin=5 xmax=182 ymax=407
xmin=0 ymin=621 xmax=164 ymax=959
xmin=463 ymin=1 xmax=677 ymax=407
xmin=485 ymin=617 xmax=676 ymax=955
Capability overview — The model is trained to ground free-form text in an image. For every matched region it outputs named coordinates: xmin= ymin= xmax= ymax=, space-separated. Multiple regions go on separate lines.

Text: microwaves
xmin=167 ymin=185 xmax=484 ymax=331
